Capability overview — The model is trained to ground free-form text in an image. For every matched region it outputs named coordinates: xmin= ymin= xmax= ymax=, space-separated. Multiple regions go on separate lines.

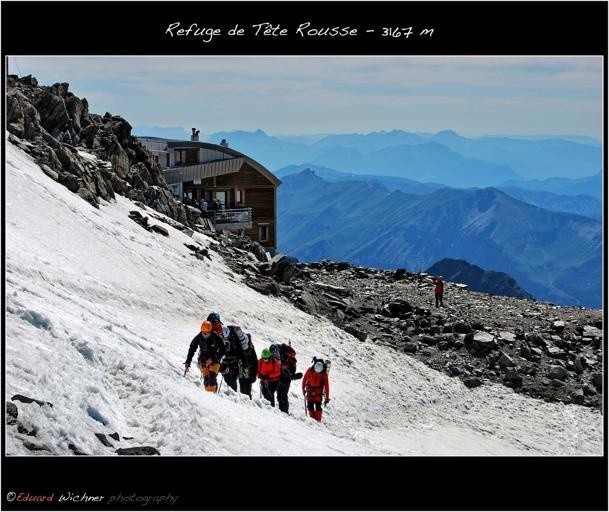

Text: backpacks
xmin=280 ymin=343 xmax=296 ymax=373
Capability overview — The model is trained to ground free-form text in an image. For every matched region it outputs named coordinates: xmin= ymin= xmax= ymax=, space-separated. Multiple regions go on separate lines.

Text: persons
xmin=275 ymin=344 xmax=292 ymax=414
xmin=433 ymin=275 xmax=444 ymax=307
xmin=256 ymin=348 xmax=280 ymax=408
xmin=184 ymin=320 xmax=226 ymax=394
xmin=238 ymin=343 xmax=258 ymax=401
xmin=301 ymin=361 xmax=329 ymax=422
xmin=220 ymin=325 xmax=243 ymax=390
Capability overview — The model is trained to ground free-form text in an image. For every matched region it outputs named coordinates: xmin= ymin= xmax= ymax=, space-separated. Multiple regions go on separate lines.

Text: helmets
xmin=314 ymin=361 xmax=324 ymax=373
xmin=208 ymin=312 xmax=220 ymax=323
xmin=262 ymin=348 xmax=271 ymax=359
xmin=201 ymin=320 xmax=212 ymax=332
